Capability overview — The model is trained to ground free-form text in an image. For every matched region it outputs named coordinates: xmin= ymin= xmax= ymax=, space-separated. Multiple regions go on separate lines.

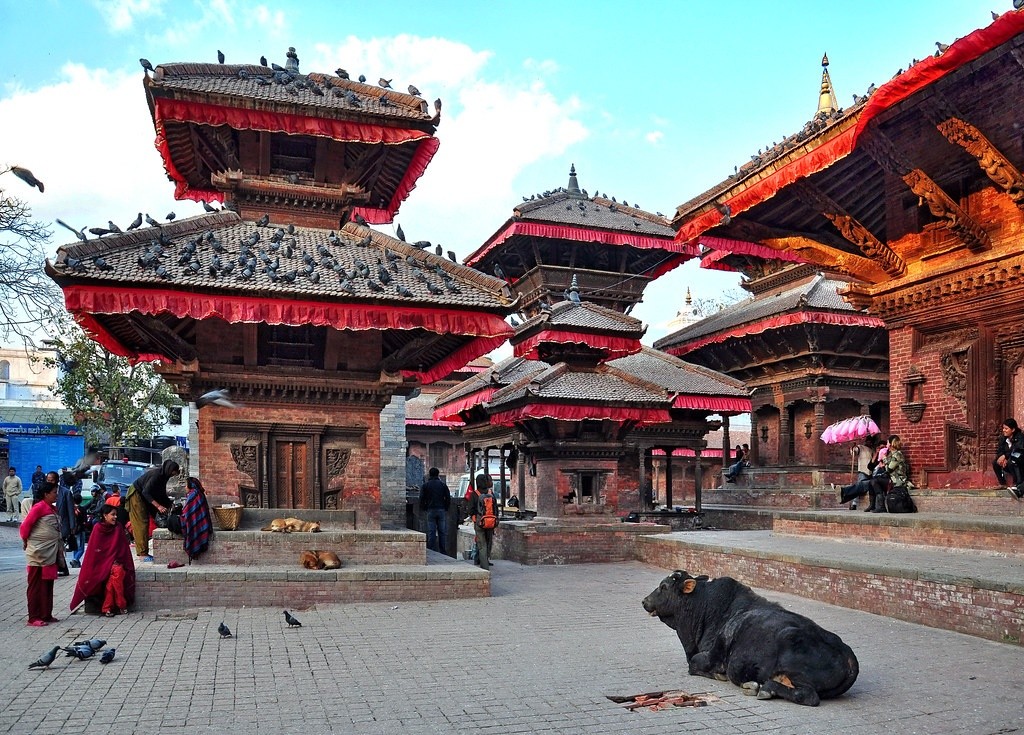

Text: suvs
xmin=91 ymin=458 xmax=157 ymax=508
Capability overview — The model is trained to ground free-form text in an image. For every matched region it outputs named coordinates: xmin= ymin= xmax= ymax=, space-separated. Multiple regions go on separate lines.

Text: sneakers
xmin=6 ymin=518 xmax=11 ymax=522
xmin=14 ymin=519 xmax=17 ymax=522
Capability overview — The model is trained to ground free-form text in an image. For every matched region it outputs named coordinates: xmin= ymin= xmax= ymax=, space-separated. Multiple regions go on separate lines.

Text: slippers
xmin=140 ymin=556 xmax=153 ymax=562
xmin=167 ymin=561 xmax=185 ymax=568
xmin=27 ymin=618 xmax=48 ymax=626
xmin=45 ymin=616 xmax=59 ymax=621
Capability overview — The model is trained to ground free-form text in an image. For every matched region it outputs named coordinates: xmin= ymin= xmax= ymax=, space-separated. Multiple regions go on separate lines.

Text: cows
xmin=642 ymin=569 xmax=860 ymax=707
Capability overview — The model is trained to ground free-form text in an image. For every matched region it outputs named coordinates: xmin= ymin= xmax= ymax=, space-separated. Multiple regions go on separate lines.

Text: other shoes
xmin=993 ymin=484 xmax=1006 ymax=490
xmin=70 ymin=560 xmax=80 ymax=567
xmin=831 ymin=483 xmax=843 ymax=503
xmin=120 ymin=608 xmax=128 ymax=614
xmin=1007 ymin=487 xmax=1023 ymax=500
xmin=488 ymin=562 xmax=493 ymax=566
xmin=107 ymin=612 xmax=114 ymax=617
xmin=849 ymin=504 xmax=856 ymax=509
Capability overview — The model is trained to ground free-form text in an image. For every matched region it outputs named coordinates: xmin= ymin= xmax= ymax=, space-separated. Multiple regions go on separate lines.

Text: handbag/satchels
xmin=155 ymin=512 xmax=167 ymax=527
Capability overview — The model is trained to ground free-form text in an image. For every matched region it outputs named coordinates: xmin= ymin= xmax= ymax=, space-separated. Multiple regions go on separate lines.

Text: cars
xmin=67 ymin=465 xmax=102 ymax=500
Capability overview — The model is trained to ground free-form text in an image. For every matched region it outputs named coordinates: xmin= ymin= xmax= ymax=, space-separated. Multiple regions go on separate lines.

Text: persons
xmin=98 ymin=483 xmax=111 ymax=501
xmin=2 ymin=467 xmax=23 ymax=522
xmin=126 ymin=460 xmax=179 ymax=556
xmin=115 ymin=496 xmax=130 ymax=527
xmin=59 ymin=467 xmax=70 ymax=489
xmin=179 ymin=477 xmax=213 ymax=560
xmin=835 ymin=435 xmax=907 ymax=514
xmin=72 ymin=479 xmax=82 ymax=496
xmin=105 ymin=485 xmax=121 ymax=506
xmin=70 ymin=494 xmax=88 ymax=568
xmin=724 ymin=444 xmax=750 ymax=483
xmin=849 ymin=435 xmax=876 ymax=510
xmin=991 ymin=418 xmax=1024 ymax=500
xmin=81 ymin=484 xmax=105 ymax=525
xmin=19 ymin=482 xmax=62 ymax=626
xmin=47 ymin=471 xmax=75 ymax=576
xmin=32 ymin=466 xmax=46 ymax=484
xmin=468 ymin=474 xmax=499 ymax=571
xmin=419 ymin=467 xmax=451 ymax=555
xmin=70 ymin=504 xmax=136 ymax=617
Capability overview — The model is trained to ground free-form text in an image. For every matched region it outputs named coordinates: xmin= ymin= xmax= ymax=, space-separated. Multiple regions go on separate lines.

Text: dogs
xmin=261 ymin=518 xmax=323 ymax=533
xmin=301 ymin=549 xmax=342 ymax=571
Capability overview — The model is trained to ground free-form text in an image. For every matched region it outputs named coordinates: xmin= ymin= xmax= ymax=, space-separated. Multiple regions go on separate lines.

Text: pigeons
xmin=218 ymin=623 xmax=232 ymax=639
xmin=138 ymin=49 xmax=422 ymax=107
xmin=28 ymin=645 xmax=61 ymax=670
xmin=99 ymin=648 xmax=116 ymax=664
xmin=55 ymin=198 xmax=506 ymax=297
xmin=727 ymin=0 xmax=1024 ymax=181
xmin=523 ymin=186 xmax=665 ymax=227
xmin=280 ymin=610 xmax=302 ymax=628
xmin=712 ymin=199 xmax=731 ymax=226
xmin=62 ymin=639 xmax=107 ymax=661
xmin=194 ymin=387 xmax=239 ymax=409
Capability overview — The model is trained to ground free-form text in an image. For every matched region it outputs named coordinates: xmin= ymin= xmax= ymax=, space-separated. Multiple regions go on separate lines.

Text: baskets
xmin=213 ymin=504 xmax=244 ymax=531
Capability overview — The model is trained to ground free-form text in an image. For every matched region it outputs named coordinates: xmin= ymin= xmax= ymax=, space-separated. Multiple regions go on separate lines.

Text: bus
xmin=454 ymin=465 xmax=511 ymax=505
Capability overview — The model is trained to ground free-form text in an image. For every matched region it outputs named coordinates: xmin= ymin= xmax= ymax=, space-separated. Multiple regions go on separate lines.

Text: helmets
xmin=91 ymin=485 xmax=101 ymax=491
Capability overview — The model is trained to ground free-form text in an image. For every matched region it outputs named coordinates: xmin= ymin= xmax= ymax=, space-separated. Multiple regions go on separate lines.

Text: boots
xmin=863 ymin=495 xmax=876 ymax=512
xmin=873 ymin=493 xmax=887 ymax=513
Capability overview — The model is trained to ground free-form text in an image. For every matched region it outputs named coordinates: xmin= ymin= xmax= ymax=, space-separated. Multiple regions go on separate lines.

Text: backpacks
xmin=72 ymin=507 xmax=83 ymax=534
xmin=473 ymin=489 xmax=497 ymax=529
xmin=887 ymin=488 xmax=914 ymax=512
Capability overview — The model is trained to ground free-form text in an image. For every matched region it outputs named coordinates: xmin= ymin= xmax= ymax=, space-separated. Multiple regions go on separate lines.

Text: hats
xmin=62 ymin=466 xmax=67 ymax=470
xmin=865 ymin=436 xmax=873 ymax=441
